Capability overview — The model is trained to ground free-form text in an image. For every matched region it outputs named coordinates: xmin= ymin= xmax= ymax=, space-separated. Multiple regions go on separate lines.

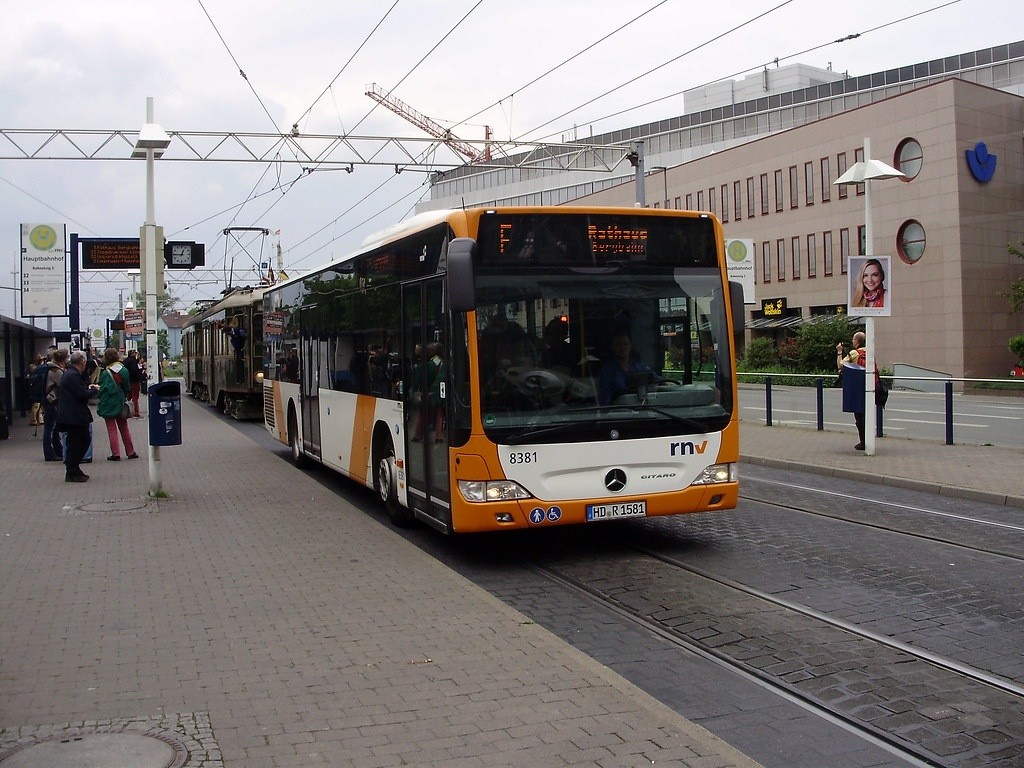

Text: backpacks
xmin=855 ymin=349 xmax=879 ymax=386
xmin=30 ymin=363 xmax=64 ymax=405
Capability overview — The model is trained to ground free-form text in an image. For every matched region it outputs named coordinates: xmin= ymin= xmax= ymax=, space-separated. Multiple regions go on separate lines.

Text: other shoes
xmin=55 ymin=457 xmax=63 ymax=461
xmin=106 ymin=454 xmax=120 ymax=461
xmin=65 ymin=464 xmax=89 ymax=482
xmin=81 ymin=458 xmax=92 ymax=463
xmin=855 ymin=442 xmax=865 ymax=450
xmin=128 ymin=451 xmax=139 ymax=459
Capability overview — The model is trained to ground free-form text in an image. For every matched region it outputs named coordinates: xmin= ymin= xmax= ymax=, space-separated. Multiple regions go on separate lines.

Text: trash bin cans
xmin=148 ymin=380 xmax=183 ymax=447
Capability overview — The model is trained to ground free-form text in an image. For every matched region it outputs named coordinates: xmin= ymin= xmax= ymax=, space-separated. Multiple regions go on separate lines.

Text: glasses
xmin=852 ymin=339 xmax=856 ymax=342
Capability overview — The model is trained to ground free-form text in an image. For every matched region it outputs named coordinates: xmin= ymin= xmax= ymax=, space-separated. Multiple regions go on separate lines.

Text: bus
xmin=261 ymin=207 xmax=747 ymax=536
xmin=181 ymin=227 xmax=274 ymax=421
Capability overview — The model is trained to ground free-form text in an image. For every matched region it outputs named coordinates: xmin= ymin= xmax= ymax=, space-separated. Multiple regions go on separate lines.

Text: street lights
xmin=831 ymin=138 xmax=907 ymax=457
xmin=129 ymin=97 xmax=172 ymax=498
xmin=650 ymin=166 xmax=668 ymax=208
xmin=127 ymin=272 xmax=141 ymax=310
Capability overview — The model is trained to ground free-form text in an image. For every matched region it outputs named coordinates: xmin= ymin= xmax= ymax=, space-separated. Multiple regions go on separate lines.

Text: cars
xmin=1010 ymin=360 xmax=1024 ymax=378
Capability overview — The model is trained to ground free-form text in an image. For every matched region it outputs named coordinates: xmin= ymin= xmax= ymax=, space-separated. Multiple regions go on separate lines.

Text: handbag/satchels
xmin=120 ymin=401 xmax=134 ymax=419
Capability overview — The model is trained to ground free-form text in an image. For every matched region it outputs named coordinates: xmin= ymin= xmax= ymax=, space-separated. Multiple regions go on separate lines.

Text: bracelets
xmin=837 ymin=354 xmax=842 ymax=355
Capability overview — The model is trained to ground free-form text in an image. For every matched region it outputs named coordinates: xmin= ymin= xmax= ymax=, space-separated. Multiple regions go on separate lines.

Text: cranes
xmin=365 ymin=81 xmax=491 ymax=162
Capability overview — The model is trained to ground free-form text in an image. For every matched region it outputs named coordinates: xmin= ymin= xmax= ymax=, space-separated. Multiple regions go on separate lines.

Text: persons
xmin=22 ymin=345 xmax=165 ymax=483
xmin=276 ymin=348 xmax=298 ymax=383
xmin=222 ymin=326 xmax=246 ymax=385
xmin=336 ymin=342 xmax=448 ymax=443
xmin=479 ymin=315 xmax=569 ymax=374
xmin=853 ymin=260 xmax=887 ymax=307
xmin=600 ymin=332 xmax=661 ymax=405
xmin=836 ymin=332 xmax=865 ymax=450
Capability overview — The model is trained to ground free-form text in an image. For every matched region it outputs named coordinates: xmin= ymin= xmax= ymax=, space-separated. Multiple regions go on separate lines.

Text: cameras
xmin=841 ymin=343 xmax=850 ymax=347
